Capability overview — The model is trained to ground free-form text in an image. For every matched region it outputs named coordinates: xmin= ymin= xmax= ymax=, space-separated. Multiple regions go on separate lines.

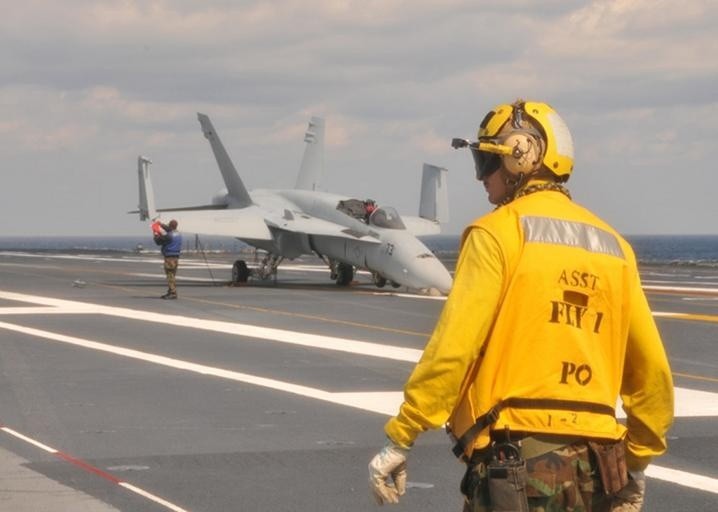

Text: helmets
xmin=468 ymin=100 xmax=574 ymax=183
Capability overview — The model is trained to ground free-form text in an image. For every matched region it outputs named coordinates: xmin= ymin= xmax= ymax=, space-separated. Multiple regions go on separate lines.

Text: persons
xmin=154 ymin=218 xmax=181 ymax=299
xmin=360 ymin=198 xmax=387 ymax=225
xmin=365 ymin=97 xmax=676 ymax=510
xmin=336 ymin=262 xmax=359 ymax=285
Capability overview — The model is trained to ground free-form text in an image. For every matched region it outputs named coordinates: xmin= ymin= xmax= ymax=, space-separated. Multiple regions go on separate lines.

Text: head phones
xmin=500 ymin=104 xmax=542 ymax=179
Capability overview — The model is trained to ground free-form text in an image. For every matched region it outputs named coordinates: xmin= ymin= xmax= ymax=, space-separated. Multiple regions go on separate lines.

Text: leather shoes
xmin=161 ymin=293 xmax=176 ymax=299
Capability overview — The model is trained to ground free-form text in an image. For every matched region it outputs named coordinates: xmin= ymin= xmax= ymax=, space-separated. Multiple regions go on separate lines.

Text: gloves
xmin=608 ymin=476 xmax=646 ymax=512
xmin=368 ymin=438 xmax=406 ymax=506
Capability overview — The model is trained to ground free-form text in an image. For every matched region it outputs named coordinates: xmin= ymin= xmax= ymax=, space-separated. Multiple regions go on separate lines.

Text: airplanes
xmin=128 ymin=113 xmax=454 ymax=295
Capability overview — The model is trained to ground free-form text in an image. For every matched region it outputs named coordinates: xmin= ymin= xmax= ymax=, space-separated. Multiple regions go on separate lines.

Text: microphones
xmin=451 ymin=138 xmax=523 ymax=158
